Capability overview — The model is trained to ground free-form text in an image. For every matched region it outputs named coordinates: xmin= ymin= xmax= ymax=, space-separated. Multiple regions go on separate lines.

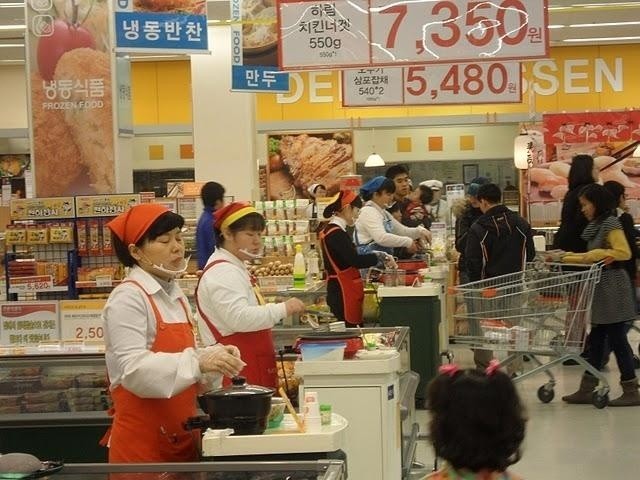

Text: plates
xmin=0 ymin=460 xmax=64 ymax=479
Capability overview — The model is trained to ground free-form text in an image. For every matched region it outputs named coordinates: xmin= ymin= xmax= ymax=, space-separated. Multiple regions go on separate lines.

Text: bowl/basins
xmin=265 ymin=397 xmax=287 ymax=429
xmin=533 ymin=329 xmax=557 ymax=346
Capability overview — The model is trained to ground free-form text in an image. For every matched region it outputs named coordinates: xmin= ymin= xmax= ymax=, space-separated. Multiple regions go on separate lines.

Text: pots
xmin=379 ymin=269 xmax=418 ymax=285
xmin=295 ymin=328 xmax=362 ymax=356
xmin=393 ymin=255 xmax=428 ymax=273
xmin=182 ymin=375 xmax=278 ymax=434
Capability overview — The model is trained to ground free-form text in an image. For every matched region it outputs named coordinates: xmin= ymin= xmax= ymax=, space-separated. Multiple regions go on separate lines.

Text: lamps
xmin=364 ymin=126 xmax=386 ymax=168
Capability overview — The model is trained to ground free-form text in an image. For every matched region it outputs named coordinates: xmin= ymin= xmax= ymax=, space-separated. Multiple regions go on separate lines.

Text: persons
xmin=101 ymin=203 xmax=248 ymax=480
xmin=454 ymin=155 xmax=639 ymax=359
xmin=304 ymin=183 xmax=327 ymax=218
xmin=195 ymin=203 xmax=308 ymax=394
xmin=563 ymin=183 xmax=640 ymax=407
xmin=414 ymin=368 xmax=527 ymax=480
xmin=191 ymin=179 xmax=226 ymax=267
xmin=322 ymin=163 xmax=450 ymax=328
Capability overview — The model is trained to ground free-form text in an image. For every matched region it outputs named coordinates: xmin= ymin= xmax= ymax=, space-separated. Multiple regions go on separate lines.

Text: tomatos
xmin=36 ymin=1 xmax=98 ymax=82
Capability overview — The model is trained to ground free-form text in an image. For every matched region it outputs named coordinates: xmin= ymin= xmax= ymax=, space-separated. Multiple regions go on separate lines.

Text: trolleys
xmin=523 ymin=253 xmax=640 ymax=361
xmin=447 ymin=249 xmax=617 ymax=411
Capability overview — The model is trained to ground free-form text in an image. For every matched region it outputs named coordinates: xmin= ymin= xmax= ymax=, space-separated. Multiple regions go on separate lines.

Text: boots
xmin=608 ymin=377 xmax=640 ymax=406
xmin=562 ymin=372 xmax=598 ymax=404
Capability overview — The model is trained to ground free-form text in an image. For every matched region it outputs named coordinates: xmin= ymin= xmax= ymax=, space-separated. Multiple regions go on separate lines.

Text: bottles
xmin=291 ymin=244 xmax=307 ymax=290
xmin=306 ymin=242 xmax=320 ymax=291
xmin=382 ymin=269 xmax=396 ymax=287
xmin=256 ymin=198 xmax=298 ymax=256
xmin=319 ymin=404 xmax=331 ymax=427
xmin=397 ymin=269 xmax=406 ymax=287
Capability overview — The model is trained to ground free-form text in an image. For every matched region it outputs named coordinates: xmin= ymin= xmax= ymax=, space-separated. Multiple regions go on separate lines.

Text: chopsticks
xmin=599 ymin=135 xmax=640 ymax=172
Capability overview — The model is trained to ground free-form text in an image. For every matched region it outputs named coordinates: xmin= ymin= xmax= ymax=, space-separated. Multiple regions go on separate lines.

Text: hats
xmin=467 ymin=176 xmax=489 ymax=196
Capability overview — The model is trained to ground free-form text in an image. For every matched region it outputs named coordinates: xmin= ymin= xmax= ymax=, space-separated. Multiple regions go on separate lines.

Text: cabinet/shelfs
xmin=243 ymin=201 xmax=324 ymax=290
xmin=5 ymin=198 xmax=77 ymax=302
xmin=170 ymin=227 xmax=205 ymax=292
xmin=19 ymin=355 xmax=116 ymax=428
xmin=75 ymin=191 xmax=139 ymax=306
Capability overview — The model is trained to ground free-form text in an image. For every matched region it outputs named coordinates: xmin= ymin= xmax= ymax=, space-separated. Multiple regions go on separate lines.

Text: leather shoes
xmin=563 ymin=354 xmax=587 ymax=366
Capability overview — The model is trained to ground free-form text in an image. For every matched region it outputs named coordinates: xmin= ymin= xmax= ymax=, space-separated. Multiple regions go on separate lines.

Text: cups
xmin=515 ymin=332 xmax=529 ymax=352
xmin=363 ymin=336 xmax=378 ymax=351
xmin=303 ymin=391 xmax=321 ymax=434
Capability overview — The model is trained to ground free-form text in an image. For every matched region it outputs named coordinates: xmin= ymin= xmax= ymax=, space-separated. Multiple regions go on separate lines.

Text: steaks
xmin=277 ymin=133 xmax=352 ymax=198
